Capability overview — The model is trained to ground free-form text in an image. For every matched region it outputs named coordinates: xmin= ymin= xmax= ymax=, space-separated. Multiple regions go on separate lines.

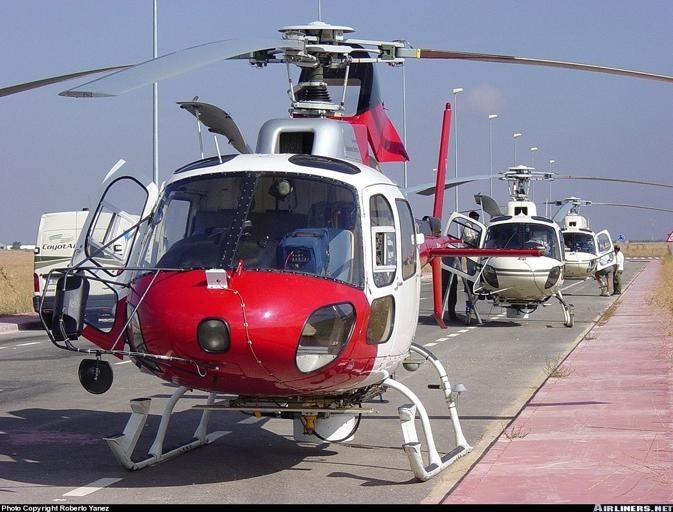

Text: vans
xmin=31 ymin=210 xmax=171 ymax=324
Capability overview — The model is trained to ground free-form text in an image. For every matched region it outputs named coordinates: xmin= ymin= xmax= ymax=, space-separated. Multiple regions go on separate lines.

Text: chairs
xmin=185 ymin=206 xmax=308 ymax=246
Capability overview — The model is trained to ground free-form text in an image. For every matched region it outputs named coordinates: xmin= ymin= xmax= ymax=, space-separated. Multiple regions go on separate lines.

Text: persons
xmin=594 ymin=243 xmax=612 ymax=297
xmin=610 ymin=244 xmax=624 ymax=295
xmin=440 ymin=240 xmax=460 ymax=322
xmin=459 ymin=211 xmax=480 ymax=293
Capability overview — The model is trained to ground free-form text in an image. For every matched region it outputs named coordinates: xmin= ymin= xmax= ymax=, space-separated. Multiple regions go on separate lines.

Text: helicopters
xmin=0 ymin=21 xmax=670 ymax=481
xmin=450 ymin=196 xmax=671 ymax=298
xmin=400 ymin=163 xmax=671 ymax=327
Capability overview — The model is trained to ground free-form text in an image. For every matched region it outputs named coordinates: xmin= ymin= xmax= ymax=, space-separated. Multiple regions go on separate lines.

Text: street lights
xmin=488 ymin=113 xmax=498 ymax=218
xmin=512 ymin=132 xmax=522 ymax=167
xmin=451 ymin=87 xmax=464 ymax=246
xmin=529 ymin=147 xmax=538 ymax=201
xmin=548 ymin=159 xmax=555 ymax=219
